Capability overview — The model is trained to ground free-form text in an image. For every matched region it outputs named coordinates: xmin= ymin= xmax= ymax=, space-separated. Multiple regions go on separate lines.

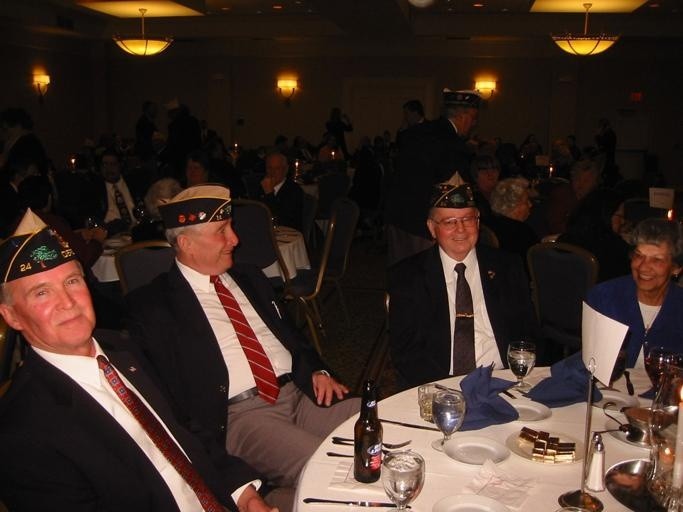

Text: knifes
xmin=624 ymin=369 xmax=635 ymax=396
xmin=502 ymin=391 xmax=516 ymax=399
xmin=377 ymin=418 xmax=440 ymax=432
xmin=303 ymin=496 xmax=413 ymax=510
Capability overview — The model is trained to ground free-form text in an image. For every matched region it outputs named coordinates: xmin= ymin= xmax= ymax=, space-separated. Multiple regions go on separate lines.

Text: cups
xmin=417 ymin=384 xmax=446 ymax=423
xmin=643 ymin=342 xmax=683 ymax=512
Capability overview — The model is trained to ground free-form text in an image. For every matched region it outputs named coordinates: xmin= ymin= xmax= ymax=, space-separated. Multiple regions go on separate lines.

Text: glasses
xmin=425 ymin=214 xmax=479 ymax=230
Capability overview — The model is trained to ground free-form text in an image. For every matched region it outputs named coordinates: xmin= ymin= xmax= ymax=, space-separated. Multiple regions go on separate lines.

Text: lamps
xmin=76 ymin=0 xmax=203 ymax=55
xmin=529 ymin=0 xmax=648 ymax=57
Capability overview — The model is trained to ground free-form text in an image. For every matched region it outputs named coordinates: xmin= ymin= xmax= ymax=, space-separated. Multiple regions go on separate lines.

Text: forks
xmin=327 ymin=436 xmax=413 ymax=461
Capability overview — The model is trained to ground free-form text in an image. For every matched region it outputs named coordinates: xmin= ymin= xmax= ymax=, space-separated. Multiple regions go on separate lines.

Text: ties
xmin=96 ymin=354 xmax=228 ymax=512
xmin=209 ymin=274 xmax=282 ymax=408
xmin=452 ymin=263 xmax=475 ymax=377
xmin=112 ymin=183 xmax=133 ymax=226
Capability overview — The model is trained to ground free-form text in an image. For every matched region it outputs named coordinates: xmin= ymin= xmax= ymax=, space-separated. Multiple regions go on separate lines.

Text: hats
xmin=0 ymin=207 xmax=77 ymax=285
xmin=157 ymin=181 xmax=234 ymax=230
xmin=428 ymin=170 xmax=478 ymax=210
xmin=440 ymin=85 xmax=494 ymax=111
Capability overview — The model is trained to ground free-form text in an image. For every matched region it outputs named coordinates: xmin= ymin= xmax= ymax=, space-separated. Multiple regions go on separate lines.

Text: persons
xmin=3 ymin=90 xmax=683 ymax=512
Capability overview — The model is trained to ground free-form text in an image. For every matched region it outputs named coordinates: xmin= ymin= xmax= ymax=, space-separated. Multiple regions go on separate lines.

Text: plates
xmin=507 ymin=432 xmax=582 ymax=465
xmin=507 ymin=399 xmax=552 ymax=422
xmin=586 ymin=386 xmax=668 ymax=512
xmin=433 ymin=494 xmax=511 ymax=512
xmin=443 ymin=437 xmax=511 ymax=466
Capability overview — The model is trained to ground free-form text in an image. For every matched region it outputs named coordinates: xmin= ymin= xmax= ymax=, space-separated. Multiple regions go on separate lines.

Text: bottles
xmin=354 ymin=379 xmax=383 ymax=484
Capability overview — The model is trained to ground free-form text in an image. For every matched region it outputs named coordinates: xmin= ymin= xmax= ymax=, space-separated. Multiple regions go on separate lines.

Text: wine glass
xmin=506 ymin=341 xmax=537 ymax=391
xmin=380 ymin=450 xmax=425 ymax=512
xmin=431 ymin=389 xmax=466 ymax=451
xmin=84 ymin=204 xmax=148 ymax=252
xmin=601 ymin=349 xmax=627 ymax=391
